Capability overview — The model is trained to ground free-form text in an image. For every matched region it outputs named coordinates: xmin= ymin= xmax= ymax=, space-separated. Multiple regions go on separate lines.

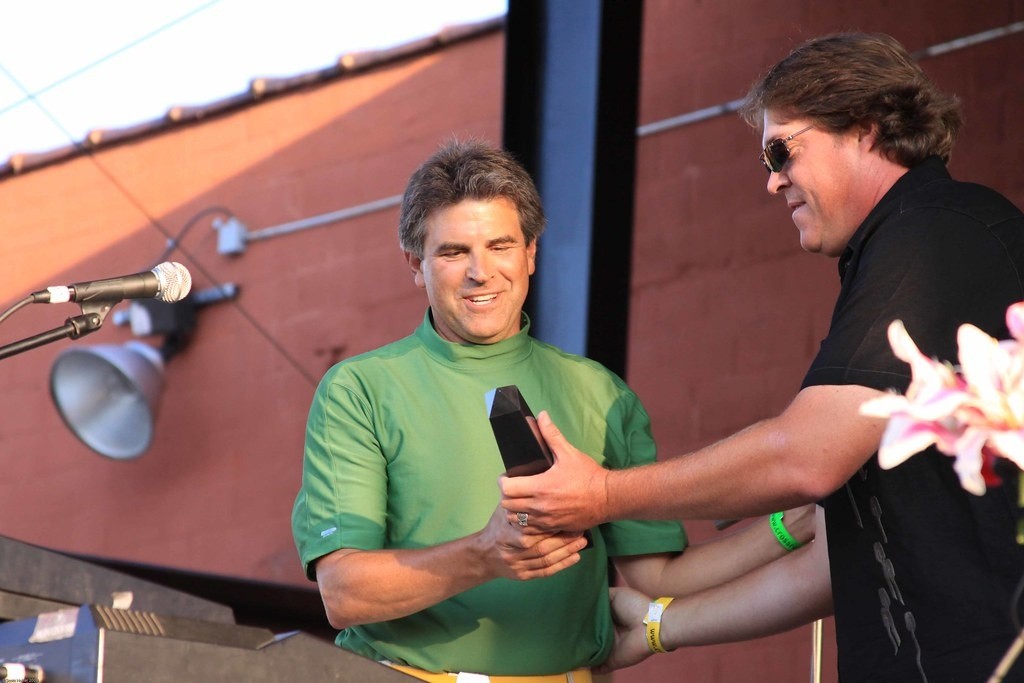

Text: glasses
xmin=761 ymin=117 xmax=823 ymax=174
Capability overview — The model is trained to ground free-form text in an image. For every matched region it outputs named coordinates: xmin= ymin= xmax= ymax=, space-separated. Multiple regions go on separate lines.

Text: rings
xmin=516 ymin=512 xmax=529 ymax=527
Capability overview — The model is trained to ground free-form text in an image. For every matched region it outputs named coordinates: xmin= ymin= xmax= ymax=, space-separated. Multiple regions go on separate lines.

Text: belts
xmin=380 ymin=656 xmax=591 ymax=683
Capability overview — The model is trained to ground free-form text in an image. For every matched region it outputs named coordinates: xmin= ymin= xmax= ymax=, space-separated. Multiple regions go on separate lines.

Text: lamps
xmin=50 ymin=294 xmax=200 ymax=459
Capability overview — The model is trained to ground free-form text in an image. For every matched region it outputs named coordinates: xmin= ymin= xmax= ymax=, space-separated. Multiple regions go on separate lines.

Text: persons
xmin=497 ymin=33 xmax=1024 ymax=683
xmin=289 ymin=140 xmax=817 ymax=683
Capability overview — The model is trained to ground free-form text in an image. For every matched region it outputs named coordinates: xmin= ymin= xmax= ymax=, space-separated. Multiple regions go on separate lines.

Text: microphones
xmin=32 ymin=261 xmax=192 ymax=305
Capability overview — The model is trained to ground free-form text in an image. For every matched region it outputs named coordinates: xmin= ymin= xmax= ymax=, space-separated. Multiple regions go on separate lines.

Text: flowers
xmin=861 ymin=302 xmax=1024 ymax=543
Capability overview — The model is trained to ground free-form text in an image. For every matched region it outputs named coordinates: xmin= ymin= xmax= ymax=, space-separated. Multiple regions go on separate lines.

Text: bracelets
xmin=768 ymin=511 xmax=802 ymax=552
xmin=642 ymin=596 xmax=678 ymax=654
